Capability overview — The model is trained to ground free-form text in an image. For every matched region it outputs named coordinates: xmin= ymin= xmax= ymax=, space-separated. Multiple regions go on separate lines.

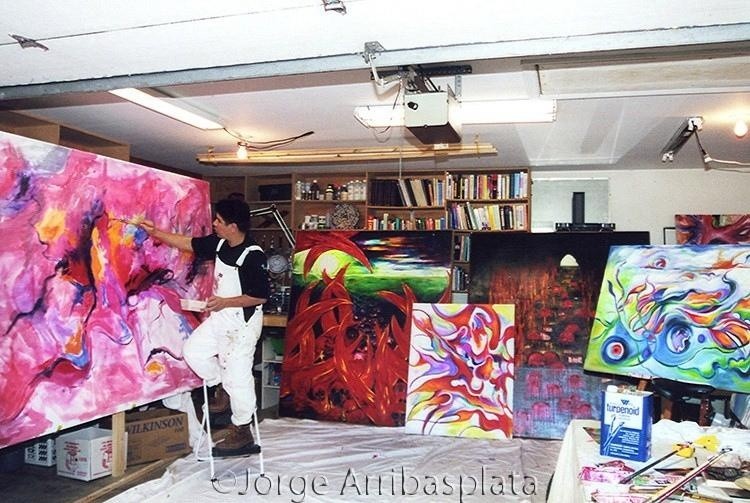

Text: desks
xmin=545 ymin=418 xmax=750 ymax=503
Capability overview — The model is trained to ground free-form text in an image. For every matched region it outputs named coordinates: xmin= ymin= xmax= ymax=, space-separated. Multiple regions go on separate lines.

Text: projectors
xmin=403 ymin=92 xmax=463 ymax=145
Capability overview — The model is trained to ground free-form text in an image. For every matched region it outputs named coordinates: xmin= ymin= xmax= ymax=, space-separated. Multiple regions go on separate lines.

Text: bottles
xmin=294 ymin=179 xmax=367 ymax=229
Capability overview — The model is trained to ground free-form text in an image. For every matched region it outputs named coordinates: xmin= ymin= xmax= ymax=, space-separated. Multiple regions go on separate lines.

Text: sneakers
xmin=215 ymin=423 xmax=255 ymax=452
xmin=209 ymin=388 xmax=230 ymax=414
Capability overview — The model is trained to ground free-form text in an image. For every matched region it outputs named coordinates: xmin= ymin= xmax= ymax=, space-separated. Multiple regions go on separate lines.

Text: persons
xmin=139 ymin=198 xmax=268 ymax=451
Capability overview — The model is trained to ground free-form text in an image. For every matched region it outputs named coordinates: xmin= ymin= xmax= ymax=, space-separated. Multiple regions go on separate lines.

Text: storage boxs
xmin=55 ymin=425 xmax=129 ymax=483
xmin=124 ymin=408 xmax=190 ymax=467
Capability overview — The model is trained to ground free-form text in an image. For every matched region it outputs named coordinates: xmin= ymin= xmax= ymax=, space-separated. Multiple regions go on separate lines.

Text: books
xmin=450 ymin=234 xmax=471 ymax=291
xmin=368 ymin=172 xmax=528 ymax=231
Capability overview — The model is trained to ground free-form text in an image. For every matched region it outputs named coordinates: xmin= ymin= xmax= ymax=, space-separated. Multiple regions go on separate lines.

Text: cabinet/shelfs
xmin=261 ymin=334 xmax=284 ymax=411
xmin=203 ymin=166 xmax=532 ymax=327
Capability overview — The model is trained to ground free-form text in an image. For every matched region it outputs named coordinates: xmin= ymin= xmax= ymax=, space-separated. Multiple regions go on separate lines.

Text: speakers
xmin=572 ymin=192 xmax=584 ymax=223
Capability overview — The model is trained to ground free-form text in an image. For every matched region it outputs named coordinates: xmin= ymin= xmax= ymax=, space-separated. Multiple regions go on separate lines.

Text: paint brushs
xmin=618 ymin=444 xmax=688 ymax=484
xmin=642 ymin=447 xmax=733 ymax=503
xmin=113 ymin=219 xmax=155 ymax=227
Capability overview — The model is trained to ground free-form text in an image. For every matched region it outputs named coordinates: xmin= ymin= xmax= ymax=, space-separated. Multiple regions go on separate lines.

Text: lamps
xmin=108 ymin=87 xmax=223 ymax=131
xmin=354 ymin=98 xmax=557 ymax=128
